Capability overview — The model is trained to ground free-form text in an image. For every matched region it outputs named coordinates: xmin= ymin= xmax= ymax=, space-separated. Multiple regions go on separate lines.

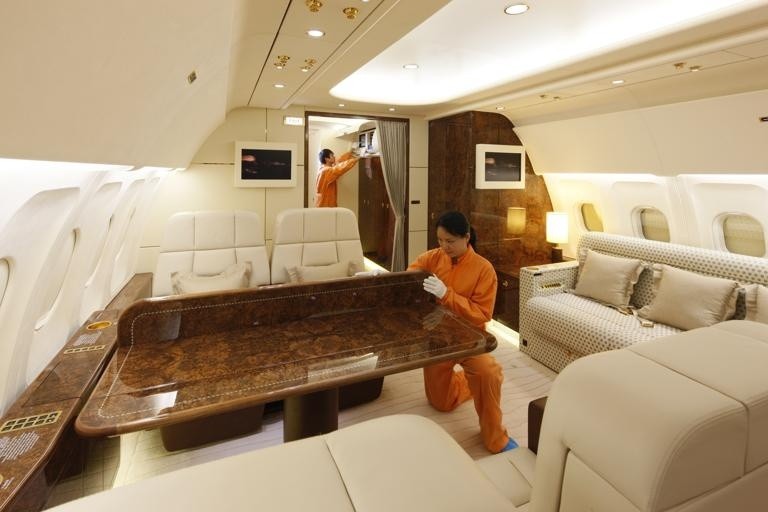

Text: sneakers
xmin=500 ymin=438 xmax=518 ymax=452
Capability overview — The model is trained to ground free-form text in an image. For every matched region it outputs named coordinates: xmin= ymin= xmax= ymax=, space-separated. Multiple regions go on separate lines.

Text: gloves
xmin=423 ymin=273 xmax=446 ymax=300
xmin=421 ymin=308 xmax=446 ymax=330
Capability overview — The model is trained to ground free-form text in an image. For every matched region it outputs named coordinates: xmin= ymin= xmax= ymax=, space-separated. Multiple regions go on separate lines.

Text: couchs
xmin=153 ymin=209 xmax=270 ymax=451
xmin=518 ymin=228 xmax=768 ymax=376
xmin=42 ymin=321 xmax=767 ymax=511
xmin=271 ymin=206 xmax=385 ymax=410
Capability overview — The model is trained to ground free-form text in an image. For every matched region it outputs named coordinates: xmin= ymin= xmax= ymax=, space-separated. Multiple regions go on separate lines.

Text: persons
xmin=314 ymin=147 xmax=363 ymax=207
xmin=407 ymin=210 xmax=509 ymax=453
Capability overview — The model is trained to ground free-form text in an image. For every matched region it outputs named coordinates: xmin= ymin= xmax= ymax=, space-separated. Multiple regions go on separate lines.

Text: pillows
xmin=745 ymin=283 xmax=767 ymax=319
xmin=641 ymin=264 xmax=740 ymax=335
xmin=567 ymin=245 xmax=645 ymax=317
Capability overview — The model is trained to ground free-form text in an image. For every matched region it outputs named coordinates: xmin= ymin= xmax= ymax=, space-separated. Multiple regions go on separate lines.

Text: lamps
xmin=545 ymin=211 xmax=570 ymax=263
xmin=506 ymin=206 xmax=527 ymax=264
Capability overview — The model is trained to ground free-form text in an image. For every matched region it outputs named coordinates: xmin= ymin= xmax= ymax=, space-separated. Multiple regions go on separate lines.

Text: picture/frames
xmin=234 ymin=141 xmax=298 ymax=187
xmin=474 ymin=144 xmax=526 ymax=189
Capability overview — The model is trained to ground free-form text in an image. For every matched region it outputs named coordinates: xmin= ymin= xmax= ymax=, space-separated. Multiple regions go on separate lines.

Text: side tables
xmin=481 ymin=260 xmax=552 ymax=323
